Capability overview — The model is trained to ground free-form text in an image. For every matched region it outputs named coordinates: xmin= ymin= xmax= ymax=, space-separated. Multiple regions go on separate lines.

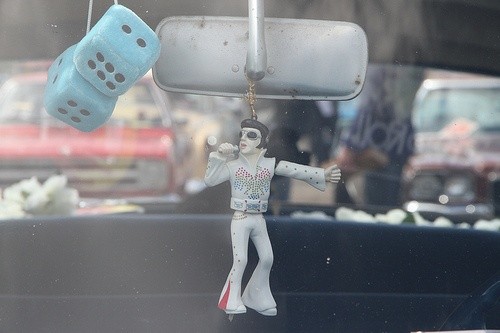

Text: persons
xmin=203 ymin=119 xmax=343 ymax=317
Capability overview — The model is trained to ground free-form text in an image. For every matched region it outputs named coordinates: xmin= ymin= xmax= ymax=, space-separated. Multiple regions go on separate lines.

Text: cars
xmin=2 ymin=70 xmax=178 ymax=200
xmin=325 ymin=72 xmax=427 ymax=203
xmin=398 ymin=81 xmax=500 ymax=220
